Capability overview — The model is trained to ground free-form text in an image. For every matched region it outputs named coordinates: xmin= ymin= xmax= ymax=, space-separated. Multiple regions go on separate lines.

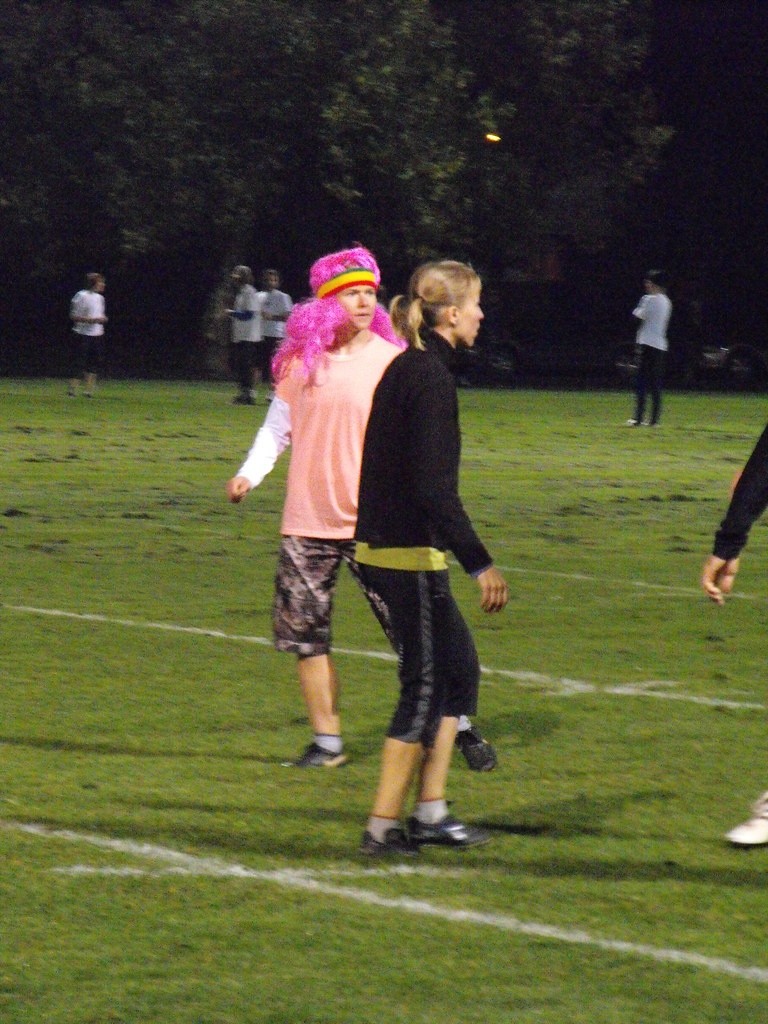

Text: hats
xmin=639 ymin=267 xmax=669 ymax=287
xmin=231 ymin=265 xmax=255 ymax=282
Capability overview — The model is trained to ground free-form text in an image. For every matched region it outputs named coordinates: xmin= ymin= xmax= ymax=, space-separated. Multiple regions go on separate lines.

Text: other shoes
xmin=726 ymin=792 xmax=768 ymax=844
xmin=234 ymin=395 xmax=258 ymax=405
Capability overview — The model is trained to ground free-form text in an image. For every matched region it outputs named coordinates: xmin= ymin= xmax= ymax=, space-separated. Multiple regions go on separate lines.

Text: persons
xmin=224 ymin=265 xmax=293 ymax=404
xmin=354 ymin=262 xmax=509 ymax=853
xmin=626 ymin=270 xmax=671 ymax=427
xmin=704 ymin=425 xmax=768 ymax=844
xmin=69 ymin=274 xmax=108 ymax=397
xmin=226 ymin=247 xmax=498 ymax=772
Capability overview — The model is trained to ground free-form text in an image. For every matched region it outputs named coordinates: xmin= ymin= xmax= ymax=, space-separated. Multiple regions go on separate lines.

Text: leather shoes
xmin=405 ymin=813 xmax=493 ymax=856
xmin=359 ymin=827 xmax=424 ymax=856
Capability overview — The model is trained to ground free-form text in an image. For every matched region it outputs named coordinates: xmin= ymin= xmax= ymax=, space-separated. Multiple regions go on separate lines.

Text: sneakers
xmin=455 ymin=726 xmax=499 ymax=772
xmin=293 ymin=742 xmax=352 ymax=769
